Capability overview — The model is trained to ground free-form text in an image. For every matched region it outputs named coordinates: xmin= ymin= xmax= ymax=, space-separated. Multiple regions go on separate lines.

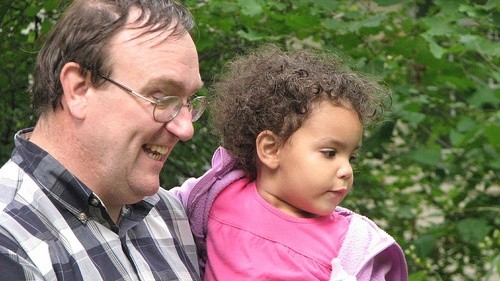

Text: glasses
xmin=90 ymin=69 xmax=210 ymax=124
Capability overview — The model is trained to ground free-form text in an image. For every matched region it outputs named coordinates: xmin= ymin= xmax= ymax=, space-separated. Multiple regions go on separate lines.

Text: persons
xmin=0 ymin=1 xmax=201 ymax=281
xmin=166 ymin=48 xmax=409 ymax=281
